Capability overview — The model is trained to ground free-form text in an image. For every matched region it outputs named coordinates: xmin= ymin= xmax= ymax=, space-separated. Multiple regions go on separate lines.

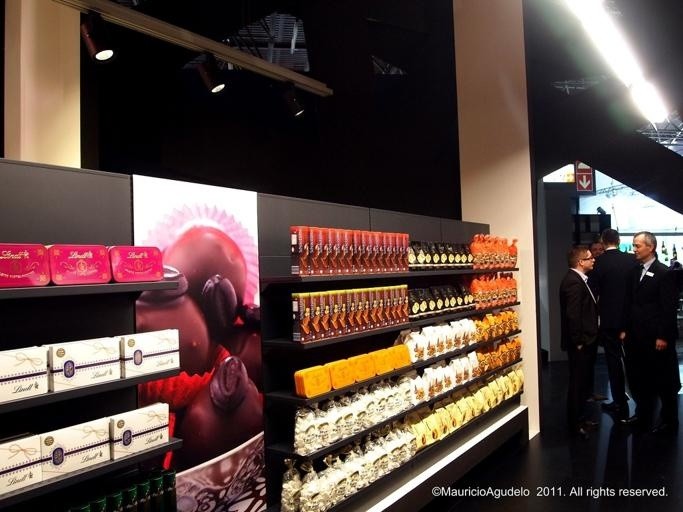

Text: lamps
xmin=79 ymin=7 xmax=113 ymax=61
xmin=282 ymin=78 xmax=304 ymax=117
xmin=193 ymin=50 xmax=225 ymax=93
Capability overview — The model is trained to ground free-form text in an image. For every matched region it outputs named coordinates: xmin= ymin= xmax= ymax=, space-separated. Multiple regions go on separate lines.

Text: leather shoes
xmin=580 ymin=418 xmax=599 ymax=427
xmin=649 ymin=420 xmax=678 ymax=436
xmin=601 ymin=402 xmax=629 ymax=414
xmin=619 ymin=414 xmax=655 ymax=426
xmin=593 ymin=393 xmax=608 ymax=402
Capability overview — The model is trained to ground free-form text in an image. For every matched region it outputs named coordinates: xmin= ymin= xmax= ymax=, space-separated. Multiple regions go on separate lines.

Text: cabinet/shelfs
xmin=258 ymin=267 xmax=524 ymax=511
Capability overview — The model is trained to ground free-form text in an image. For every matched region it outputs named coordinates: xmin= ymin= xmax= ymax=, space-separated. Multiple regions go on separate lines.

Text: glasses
xmin=582 ymin=254 xmax=594 ymax=261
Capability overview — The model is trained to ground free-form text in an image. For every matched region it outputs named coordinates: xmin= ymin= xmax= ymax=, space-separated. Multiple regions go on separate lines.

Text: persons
xmin=617 ymin=231 xmax=683 ymax=439
xmin=557 ymin=244 xmax=600 ymax=443
xmin=669 ymin=260 xmax=683 ymax=289
xmin=588 ymin=227 xmax=643 ymax=415
xmin=582 ymin=232 xmax=611 ymax=400
